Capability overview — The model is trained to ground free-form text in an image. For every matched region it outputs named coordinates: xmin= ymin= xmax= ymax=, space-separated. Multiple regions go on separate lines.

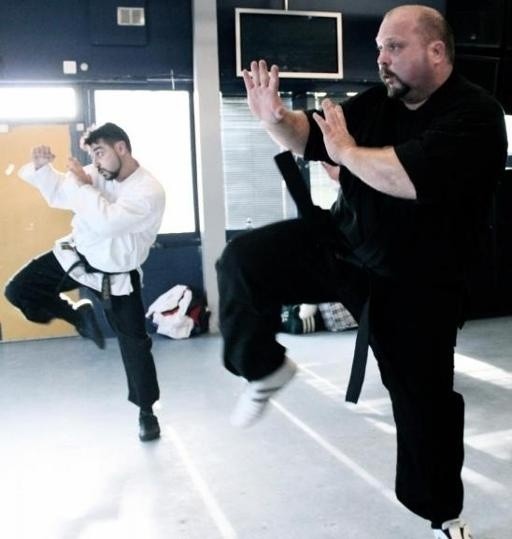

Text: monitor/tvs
xmin=234 ymin=7 xmax=344 ymax=80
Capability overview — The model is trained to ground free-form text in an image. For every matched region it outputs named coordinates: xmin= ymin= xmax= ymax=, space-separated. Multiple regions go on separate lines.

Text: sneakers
xmin=432 ymin=519 xmax=474 ymax=539
xmin=230 ymin=359 xmax=296 ymax=428
xmin=138 ymin=415 xmax=160 ymax=441
xmin=75 ymin=300 xmax=104 ymax=350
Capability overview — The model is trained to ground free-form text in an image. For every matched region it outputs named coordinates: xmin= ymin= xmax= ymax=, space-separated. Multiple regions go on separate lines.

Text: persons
xmin=216 ymin=3 xmax=507 ymax=539
xmin=4 ymin=122 xmax=166 ymax=444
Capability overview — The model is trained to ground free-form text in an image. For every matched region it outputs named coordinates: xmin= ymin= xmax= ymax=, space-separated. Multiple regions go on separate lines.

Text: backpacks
xmin=144 ymin=282 xmax=211 ymax=340
